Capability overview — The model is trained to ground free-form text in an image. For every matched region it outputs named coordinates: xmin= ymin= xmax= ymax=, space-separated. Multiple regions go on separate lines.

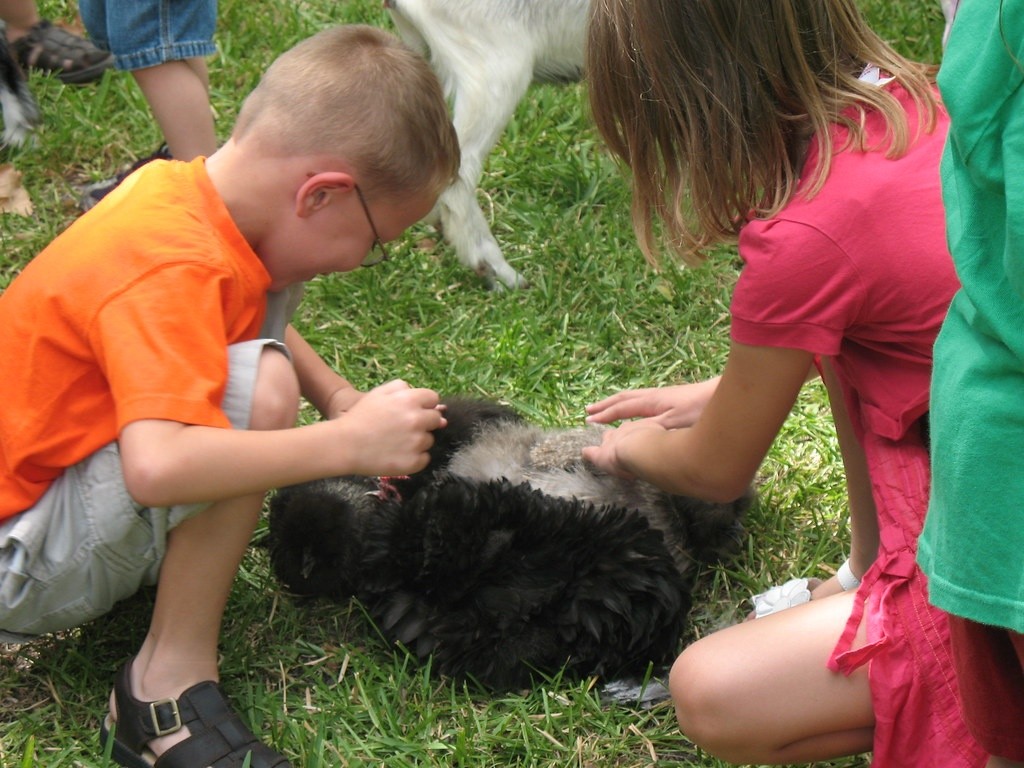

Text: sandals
xmin=750 ymin=557 xmax=864 ymax=620
xmin=100 ymin=654 xmax=294 ymax=768
xmin=7 ymin=18 xmax=114 ymax=82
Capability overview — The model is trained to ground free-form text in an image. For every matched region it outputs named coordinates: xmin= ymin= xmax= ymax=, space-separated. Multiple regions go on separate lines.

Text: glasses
xmin=307 ymin=171 xmax=390 ymax=268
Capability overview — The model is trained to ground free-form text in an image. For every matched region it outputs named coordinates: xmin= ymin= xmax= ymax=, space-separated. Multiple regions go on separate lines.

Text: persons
xmin=2 ymin=0 xmax=215 ymax=216
xmin=581 ymin=0 xmax=1023 ymax=768
xmin=0 ymin=20 xmax=460 ymax=768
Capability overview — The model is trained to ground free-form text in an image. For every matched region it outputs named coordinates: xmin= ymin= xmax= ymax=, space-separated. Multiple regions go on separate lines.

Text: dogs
xmin=271 ymin=392 xmax=748 ymax=709
xmin=386 ymin=0 xmax=602 ymax=286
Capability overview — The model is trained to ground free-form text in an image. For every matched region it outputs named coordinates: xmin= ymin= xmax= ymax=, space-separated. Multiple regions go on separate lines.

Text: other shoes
xmin=80 ymin=142 xmax=172 ymax=213
